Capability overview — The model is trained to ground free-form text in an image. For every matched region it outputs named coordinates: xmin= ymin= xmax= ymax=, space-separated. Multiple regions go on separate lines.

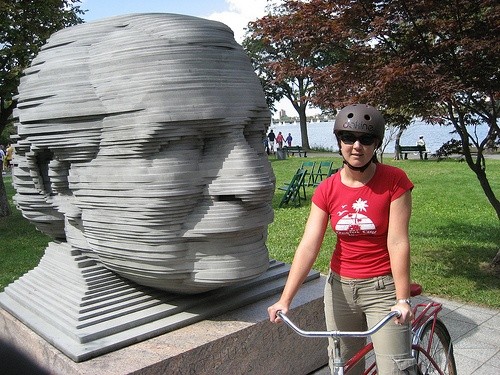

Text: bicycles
xmin=276 ymin=284 xmax=457 ymax=375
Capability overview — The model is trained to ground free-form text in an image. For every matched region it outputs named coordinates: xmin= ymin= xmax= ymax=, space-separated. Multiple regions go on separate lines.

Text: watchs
xmin=395 ymin=297 xmax=412 ymax=306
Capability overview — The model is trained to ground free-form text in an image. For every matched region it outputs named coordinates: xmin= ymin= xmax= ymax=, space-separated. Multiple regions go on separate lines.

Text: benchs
xmin=284 ymin=145 xmax=307 ymax=158
xmin=400 ymin=145 xmax=431 ymax=160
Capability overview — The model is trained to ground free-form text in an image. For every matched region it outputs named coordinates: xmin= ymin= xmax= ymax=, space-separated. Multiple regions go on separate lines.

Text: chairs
xmin=277 ymin=160 xmax=336 ymax=208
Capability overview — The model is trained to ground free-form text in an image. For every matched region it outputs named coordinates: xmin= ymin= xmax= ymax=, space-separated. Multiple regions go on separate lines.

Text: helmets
xmin=333 ymin=103 xmax=385 ymax=140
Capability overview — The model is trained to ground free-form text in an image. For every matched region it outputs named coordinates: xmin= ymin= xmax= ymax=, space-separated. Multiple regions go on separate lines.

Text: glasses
xmin=340 ymin=132 xmax=376 ymax=146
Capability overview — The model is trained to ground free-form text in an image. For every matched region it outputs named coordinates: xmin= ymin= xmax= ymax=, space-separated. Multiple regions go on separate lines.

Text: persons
xmin=264 ymin=129 xmax=292 ymax=155
xmin=0 ymin=144 xmax=13 ymax=173
xmin=417 ymin=135 xmax=426 ymax=160
xmin=7 ymin=12 xmax=277 ymax=291
xmin=267 ymin=104 xmax=419 ymax=375
